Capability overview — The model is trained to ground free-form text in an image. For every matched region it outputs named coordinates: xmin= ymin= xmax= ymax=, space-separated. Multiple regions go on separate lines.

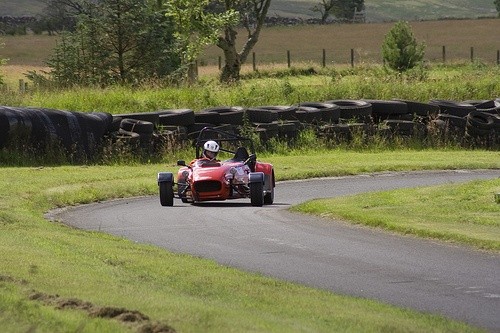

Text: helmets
xmin=203 ymin=141 xmax=220 ymax=162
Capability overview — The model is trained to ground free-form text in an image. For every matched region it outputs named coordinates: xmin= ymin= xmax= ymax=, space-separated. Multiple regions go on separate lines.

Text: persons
xmin=188 ymin=140 xmax=221 ymax=166
